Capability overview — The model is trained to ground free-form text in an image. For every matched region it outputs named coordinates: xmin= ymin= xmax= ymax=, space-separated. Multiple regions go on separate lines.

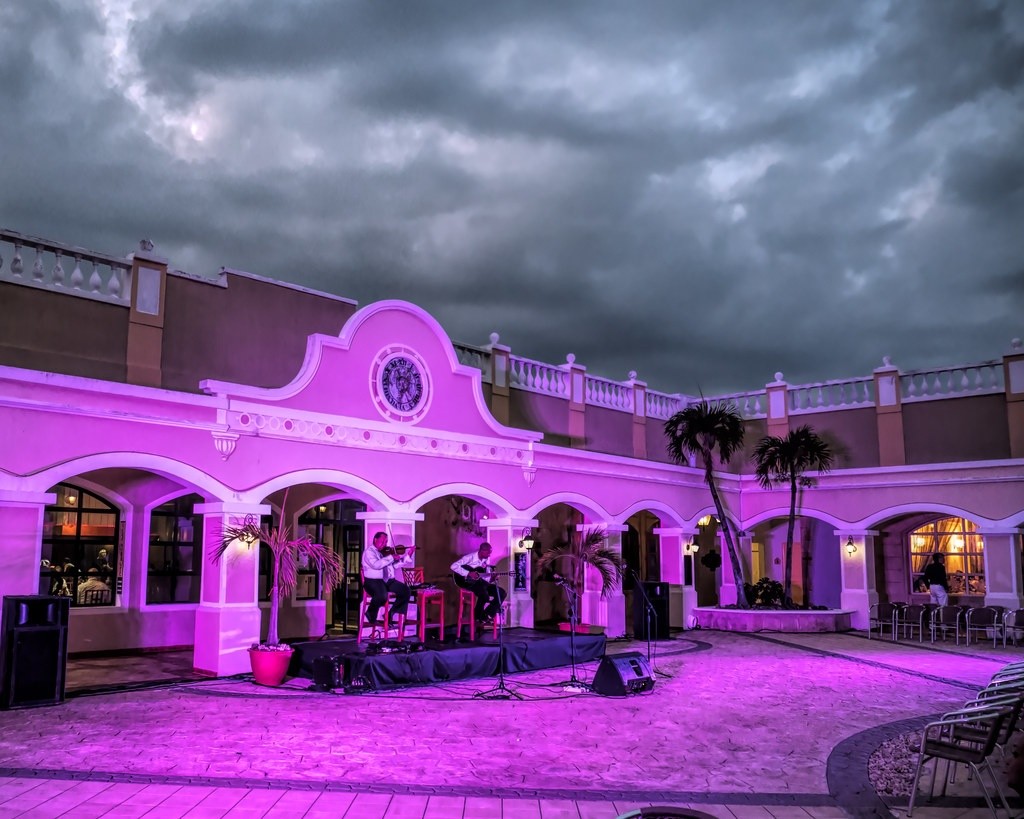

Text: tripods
xmin=630 ymin=572 xmax=672 ymax=678
xmin=474 ymin=568 xmax=524 ymax=701
xmin=550 ymin=580 xmax=592 ymax=687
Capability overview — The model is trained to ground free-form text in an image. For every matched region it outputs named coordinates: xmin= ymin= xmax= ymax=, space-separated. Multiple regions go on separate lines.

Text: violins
xmin=380 ymin=545 xmax=421 ymax=557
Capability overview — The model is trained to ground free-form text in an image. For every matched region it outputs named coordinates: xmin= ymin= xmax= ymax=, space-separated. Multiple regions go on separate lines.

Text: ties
xmin=380 ymin=555 xmax=389 ymax=583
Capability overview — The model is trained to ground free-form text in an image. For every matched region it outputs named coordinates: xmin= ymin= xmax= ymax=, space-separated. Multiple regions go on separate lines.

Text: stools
xmin=356 ymin=568 xmax=403 ymax=647
xmin=401 ymin=566 xmax=445 ymax=643
xmin=456 ymin=588 xmax=498 ymax=641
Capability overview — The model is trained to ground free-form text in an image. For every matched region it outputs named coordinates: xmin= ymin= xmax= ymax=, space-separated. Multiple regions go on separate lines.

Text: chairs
xmin=868 ymin=601 xmax=1024 ymax=649
xmin=906 ymin=660 xmax=1024 ymax=819
xmin=83 ymin=589 xmax=110 ymax=605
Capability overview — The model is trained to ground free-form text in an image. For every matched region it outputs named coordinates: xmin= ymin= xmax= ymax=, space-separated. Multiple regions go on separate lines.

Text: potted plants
xmin=533 ymin=525 xmax=626 ymax=634
xmin=206 ymin=487 xmax=345 ymax=686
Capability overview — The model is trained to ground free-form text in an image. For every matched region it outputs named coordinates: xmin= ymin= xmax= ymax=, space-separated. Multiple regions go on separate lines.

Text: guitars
xmin=453 ymin=564 xmax=516 ymax=588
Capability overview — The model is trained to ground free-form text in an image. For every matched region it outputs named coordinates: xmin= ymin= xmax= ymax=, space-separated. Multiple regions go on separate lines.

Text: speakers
xmin=0 ymin=594 xmax=71 ymax=711
xmin=590 ymin=651 xmax=656 ymax=697
xmin=634 ymin=581 xmax=670 ymax=641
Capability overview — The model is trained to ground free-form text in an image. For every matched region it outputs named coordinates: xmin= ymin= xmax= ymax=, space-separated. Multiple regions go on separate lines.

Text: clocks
xmin=376 ymin=351 xmax=429 ymax=417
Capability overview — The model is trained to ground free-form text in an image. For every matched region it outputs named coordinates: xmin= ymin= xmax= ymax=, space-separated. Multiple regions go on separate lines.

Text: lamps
xmin=518 ymin=527 xmax=533 ymax=554
xmin=238 ymin=523 xmax=254 ymax=549
xmin=845 ymin=535 xmax=858 ymax=557
xmin=697 ymin=515 xmax=711 ymax=532
xmin=685 ymin=534 xmax=699 ymax=554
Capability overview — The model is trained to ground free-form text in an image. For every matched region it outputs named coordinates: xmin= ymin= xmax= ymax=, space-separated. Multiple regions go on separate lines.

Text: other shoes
xmin=365 ymin=611 xmax=378 ymax=624
xmin=382 ymin=611 xmax=393 ymax=627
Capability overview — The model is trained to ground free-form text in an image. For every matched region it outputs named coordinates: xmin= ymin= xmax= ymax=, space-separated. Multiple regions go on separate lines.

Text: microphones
xmin=554 ymin=574 xmax=568 ymax=580
xmin=482 ymin=563 xmax=496 ymax=569
xmin=622 ymin=565 xmax=636 ymax=574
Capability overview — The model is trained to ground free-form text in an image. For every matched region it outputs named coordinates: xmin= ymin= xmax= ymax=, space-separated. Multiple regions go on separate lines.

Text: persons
xmin=97 ymin=549 xmax=113 ymax=582
xmin=915 ymin=570 xmax=985 ymax=593
xmin=64 ymin=558 xmax=74 ymax=571
xmin=52 ymin=566 xmax=70 ymax=596
xmin=923 ymin=553 xmax=948 ymax=606
xmin=361 ymin=532 xmax=417 ymax=626
xmin=78 ymin=568 xmax=111 ymax=604
xmin=450 ymin=542 xmax=507 ymax=624
